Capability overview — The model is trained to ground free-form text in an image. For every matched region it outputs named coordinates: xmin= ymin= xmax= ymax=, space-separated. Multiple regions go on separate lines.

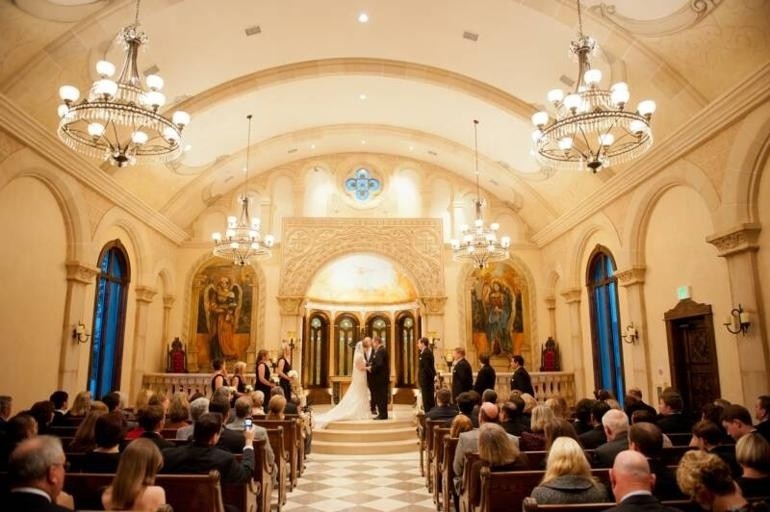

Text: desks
xmin=329 ymin=375 xmax=394 ymax=410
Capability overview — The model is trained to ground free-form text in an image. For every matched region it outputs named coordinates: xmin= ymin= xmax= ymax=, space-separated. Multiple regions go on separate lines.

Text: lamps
xmin=56 ymin=0 xmax=191 ymax=169
xmin=723 ymin=306 xmax=752 ymax=338
xmin=620 ymin=323 xmax=637 ymax=343
xmin=73 ymin=320 xmax=91 ymax=346
xmin=268 ymin=348 xmax=281 ymax=373
xmin=208 ymin=112 xmax=276 ymax=267
xmin=442 ymin=351 xmax=454 ymax=373
xmin=426 ymin=329 xmax=439 ymax=356
xmin=284 ymin=329 xmax=298 ymax=368
xmin=530 ymin=1 xmax=655 ymax=178
xmin=451 ymin=118 xmax=509 ymax=269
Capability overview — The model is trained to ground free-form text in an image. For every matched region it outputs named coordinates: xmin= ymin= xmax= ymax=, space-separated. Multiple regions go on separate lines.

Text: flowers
xmin=287 ymin=370 xmax=298 ymax=380
xmin=225 ymin=386 xmax=236 ymax=394
xmin=269 ymin=375 xmax=281 ymax=384
xmin=245 ymin=385 xmax=253 ymax=392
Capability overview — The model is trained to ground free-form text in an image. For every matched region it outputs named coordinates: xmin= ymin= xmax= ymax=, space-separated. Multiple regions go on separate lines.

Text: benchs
xmin=0 ymin=396 xmax=309 ymax=512
xmin=414 ymin=405 xmax=770 ymax=512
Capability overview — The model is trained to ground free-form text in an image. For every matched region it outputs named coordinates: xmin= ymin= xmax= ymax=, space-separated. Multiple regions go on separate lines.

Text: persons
xmin=254 ymin=349 xmax=275 ymax=412
xmin=430 ymin=387 xmax=769 ymax=511
xmin=416 ymin=338 xmax=436 ymax=415
xmin=368 ymin=336 xmax=390 ymax=420
xmin=352 ymin=341 xmax=372 ymax=422
xmin=207 ymin=277 xmax=237 ymax=360
xmin=474 ymin=353 xmax=496 ymax=396
xmin=0 ymin=385 xmax=301 ymax=511
xmin=231 ymin=361 xmax=250 ymax=406
xmin=277 ymin=341 xmax=294 ymax=403
xmin=452 ymin=347 xmax=473 ymax=406
xmin=510 ymin=355 xmax=534 ymax=398
xmin=211 ymin=357 xmax=230 ymax=393
xmin=484 ymin=280 xmax=514 ymax=359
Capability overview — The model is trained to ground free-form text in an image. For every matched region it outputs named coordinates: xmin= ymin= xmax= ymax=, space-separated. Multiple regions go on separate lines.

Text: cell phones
xmin=245 ymin=418 xmax=252 ymax=431
